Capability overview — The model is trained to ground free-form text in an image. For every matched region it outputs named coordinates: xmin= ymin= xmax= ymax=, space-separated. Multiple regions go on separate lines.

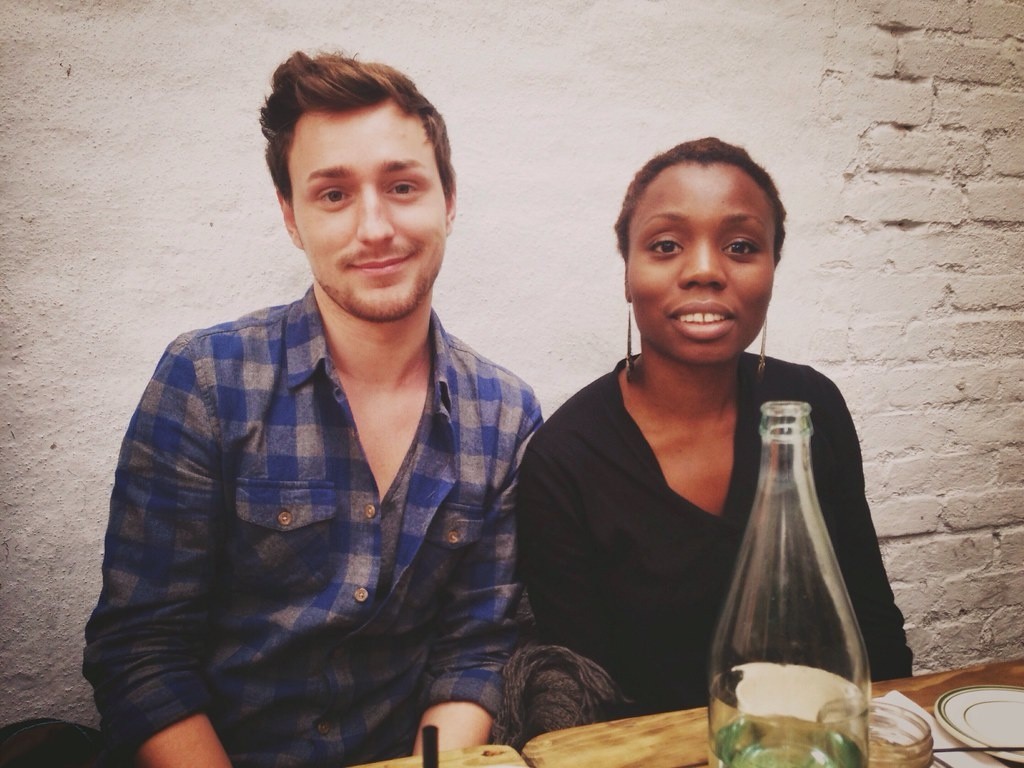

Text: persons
xmin=517 ymin=140 xmax=913 ymax=721
xmin=0 ymin=50 xmax=546 ymax=768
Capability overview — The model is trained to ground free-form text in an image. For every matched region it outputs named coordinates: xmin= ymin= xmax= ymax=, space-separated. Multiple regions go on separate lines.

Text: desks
xmin=522 ymin=658 xmax=1024 ymax=768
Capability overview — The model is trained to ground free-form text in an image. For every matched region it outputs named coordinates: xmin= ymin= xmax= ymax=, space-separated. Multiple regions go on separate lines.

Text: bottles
xmin=816 ymin=700 xmax=934 ymax=768
xmin=707 ymin=400 xmax=873 ymax=768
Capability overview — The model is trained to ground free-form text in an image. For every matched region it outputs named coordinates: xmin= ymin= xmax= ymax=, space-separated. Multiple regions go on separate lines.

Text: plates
xmin=933 ymin=684 xmax=1024 ymax=763
